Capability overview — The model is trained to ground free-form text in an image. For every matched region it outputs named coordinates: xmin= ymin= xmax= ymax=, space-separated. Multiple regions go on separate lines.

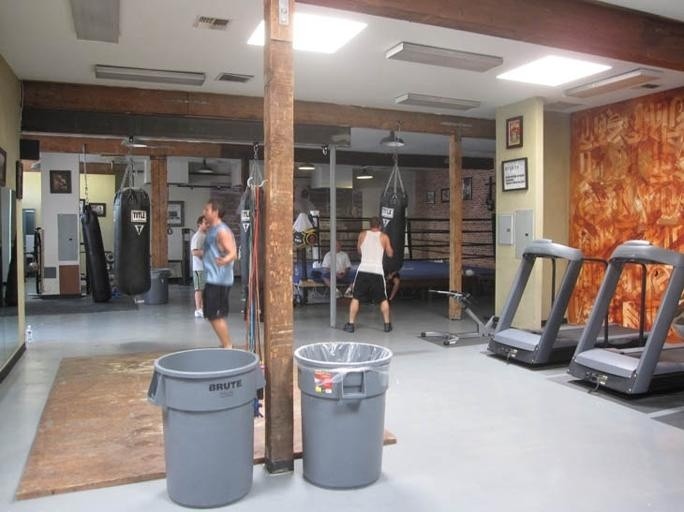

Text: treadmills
xmin=567 ymin=239 xmax=684 ymax=394
xmin=489 ymin=238 xmax=651 ymax=369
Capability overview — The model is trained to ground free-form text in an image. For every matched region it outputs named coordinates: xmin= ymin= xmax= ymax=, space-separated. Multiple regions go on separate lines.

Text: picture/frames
xmin=441 ymin=188 xmax=450 ymax=202
xmin=462 ymin=177 xmax=472 ymax=200
xmin=49 ymin=170 xmax=71 ymax=193
xmin=0 ymin=146 xmax=6 ymax=186
xmin=16 ymin=160 xmax=23 ymax=199
xmin=89 ymin=203 xmax=106 ymax=217
xmin=166 ymin=201 xmax=184 ymax=227
xmin=506 ymin=115 xmax=523 ymax=148
xmin=79 ymin=199 xmax=85 ymax=215
xmin=501 ymin=157 xmax=527 ymax=192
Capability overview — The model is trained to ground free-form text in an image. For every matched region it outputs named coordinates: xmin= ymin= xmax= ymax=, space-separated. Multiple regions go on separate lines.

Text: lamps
xmin=355 ymin=154 xmax=374 ymax=180
xmin=298 ymin=163 xmax=316 ymax=170
xmin=380 ymin=128 xmax=405 ymax=147
xmin=195 ymin=156 xmax=215 ymax=174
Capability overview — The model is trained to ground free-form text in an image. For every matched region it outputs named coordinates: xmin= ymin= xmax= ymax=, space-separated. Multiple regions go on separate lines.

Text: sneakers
xmin=384 ymin=322 xmax=393 ymax=333
xmin=334 ymin=289 xmax=354 ymax=298
xmin=342 ymin=322 xmax=354 ymax=333
xmin=194 ymin=309 xmax=206 ymax=318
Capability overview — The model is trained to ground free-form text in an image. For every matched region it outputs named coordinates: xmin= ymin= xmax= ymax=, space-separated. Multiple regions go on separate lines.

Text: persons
xmin=319 ymin=240 xmax=352 ymax=301
xmin=189 ymin=215 xmax=209 ymax=318
xmin=292 ymin=188 xmax=315 ymax=214
xmin=194 ymin=197 xmax=237 ymax=348
xmin=387 ymin=272 xmax=400 ymax=305
xmin=342 ymin=215 xmax=395 ymax=332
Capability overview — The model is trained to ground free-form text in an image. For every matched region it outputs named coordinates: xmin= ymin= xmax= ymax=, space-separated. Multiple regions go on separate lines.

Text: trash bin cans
xmin=148 ymin=348 xmax=266 ymax=509
xmin=296 ymin=342 xmax=393 ymax=489
xmin=145 ymin=269 xmax=170 ymax=304
